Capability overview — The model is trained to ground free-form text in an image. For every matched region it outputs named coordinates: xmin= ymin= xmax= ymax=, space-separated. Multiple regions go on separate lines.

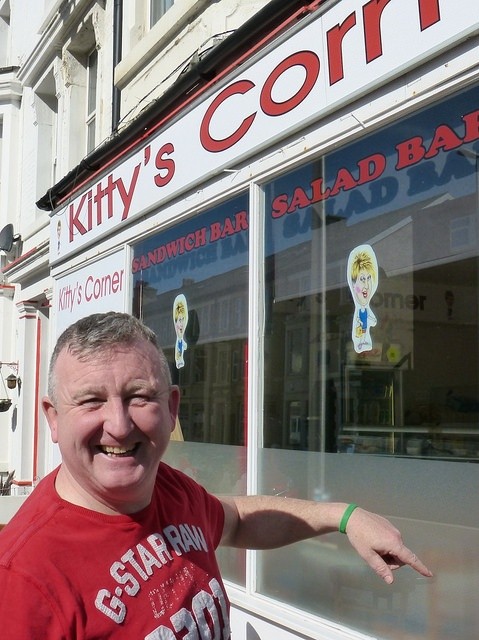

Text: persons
xmin=0 ymin=311 xmax=435 ymax=640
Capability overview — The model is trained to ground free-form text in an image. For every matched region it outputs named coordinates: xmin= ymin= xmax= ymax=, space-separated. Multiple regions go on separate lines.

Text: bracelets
xmin=339 ymin=503 xmax=359 ymax=534
